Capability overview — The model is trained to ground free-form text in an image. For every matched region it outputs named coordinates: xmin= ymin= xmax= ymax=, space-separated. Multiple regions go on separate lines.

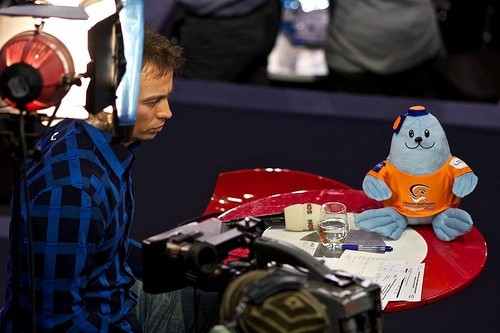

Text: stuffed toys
xmin=354 ymin=106 xmax=479 ymax=241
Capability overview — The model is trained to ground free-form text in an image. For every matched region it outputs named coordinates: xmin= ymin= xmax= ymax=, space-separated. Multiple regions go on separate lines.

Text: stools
xmin=198 ymin=168 xmax=353 ymax=223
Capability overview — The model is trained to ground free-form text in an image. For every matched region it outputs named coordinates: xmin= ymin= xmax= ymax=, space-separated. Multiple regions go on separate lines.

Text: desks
xmin=216 ymin=188 xmax=487 ymax=314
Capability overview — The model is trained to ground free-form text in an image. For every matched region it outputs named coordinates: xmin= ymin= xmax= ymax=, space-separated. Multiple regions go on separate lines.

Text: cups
xmin=316 ymin=202 xmax=350 ymax=251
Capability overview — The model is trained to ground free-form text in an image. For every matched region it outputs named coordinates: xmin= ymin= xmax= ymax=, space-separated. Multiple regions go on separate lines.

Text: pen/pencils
xmin=341 ymin=244 xmax=393 ymax=254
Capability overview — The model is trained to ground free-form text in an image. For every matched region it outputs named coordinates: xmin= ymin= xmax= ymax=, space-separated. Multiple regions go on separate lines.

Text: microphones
xmin=225 ymin=203 xmax=322 ymax=231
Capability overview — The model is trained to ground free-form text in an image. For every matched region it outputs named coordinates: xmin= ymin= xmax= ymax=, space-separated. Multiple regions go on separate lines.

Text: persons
xmin=325 ymin=0 xmax=448 ymax=100
xmin=157 ymin=0 xmax=283 ymax=86
xmin=0 ymin=29 xmax=187 ymax=333
